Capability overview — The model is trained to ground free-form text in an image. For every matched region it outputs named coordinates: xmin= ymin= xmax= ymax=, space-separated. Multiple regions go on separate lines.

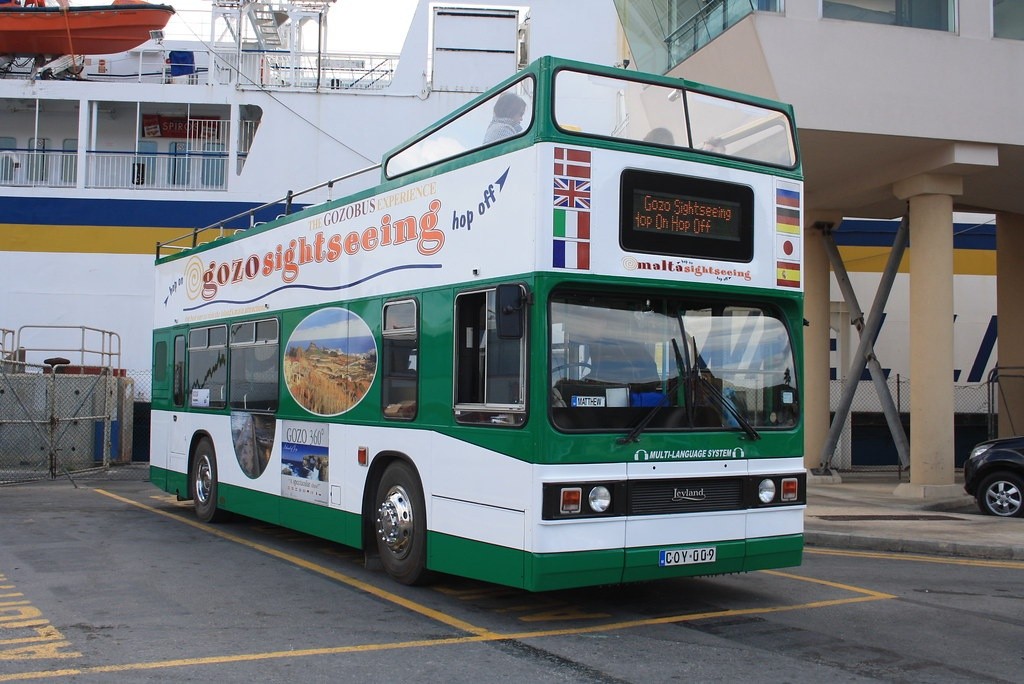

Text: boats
xmin=0 ymin=3 xmax=1023 ymax=472
xmin=0 ymin=0 xmax=176 ymax=56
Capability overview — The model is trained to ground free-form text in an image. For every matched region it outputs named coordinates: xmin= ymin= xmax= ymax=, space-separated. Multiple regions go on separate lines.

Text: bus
xmin=148 ymin=56 xmax=804 ymax=591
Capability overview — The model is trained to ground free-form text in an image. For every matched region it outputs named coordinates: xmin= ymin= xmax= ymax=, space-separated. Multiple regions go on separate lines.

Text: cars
xmin=964 ymin=437 xmax=1024 ymax=518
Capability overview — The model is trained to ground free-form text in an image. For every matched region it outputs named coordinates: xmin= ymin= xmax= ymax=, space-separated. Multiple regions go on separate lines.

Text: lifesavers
xmin=0 ymin=151 xmax=21 ymax=183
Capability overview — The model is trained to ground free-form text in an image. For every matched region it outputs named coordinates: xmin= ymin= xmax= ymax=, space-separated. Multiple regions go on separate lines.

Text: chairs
xmin=202 ymin=381 xmax=275 ymax=409
